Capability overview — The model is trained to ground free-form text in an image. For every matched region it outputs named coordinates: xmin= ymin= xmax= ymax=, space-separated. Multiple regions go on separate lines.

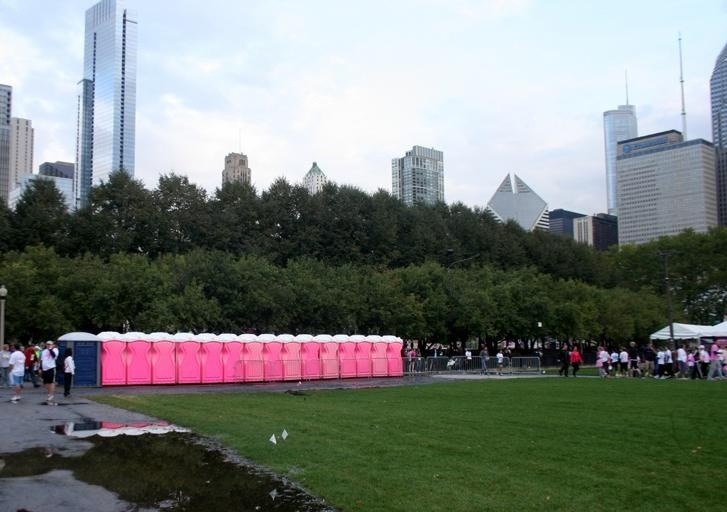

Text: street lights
xmin=0 ymin=284 xmax=8 ymax=352
xmin=657 ymin=249 xmax=676 ymax=353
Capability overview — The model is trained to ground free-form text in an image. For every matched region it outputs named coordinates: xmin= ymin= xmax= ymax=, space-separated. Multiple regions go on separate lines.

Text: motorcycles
xmin=446 ymin=358 xmax=465 ymax=369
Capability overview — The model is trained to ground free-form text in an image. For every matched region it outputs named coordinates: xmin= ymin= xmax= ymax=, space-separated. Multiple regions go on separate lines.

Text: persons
xmin=479 ymin=346 xmax=490 ymax=375
xmin=447 ymin=359 xmax=455 ymax=369
xmin=0 ymin=340 xmax=76 ymax=406
xmin=404 ymin=341 xmax=421 ymax=373
xmin=569 ymin=346 xmax=584 ymax=377
xmin=556 ymin=343 xmax=570 ymax=377
xmin=465 ymin=348 xmax=472 ymax=368
xmin=595 ymin=342 xmax=727 ymax=380
xmin=496 ymin=349 xmax=504 ymax=375
xmin=503 ymin=348 xmax=512 ymax=368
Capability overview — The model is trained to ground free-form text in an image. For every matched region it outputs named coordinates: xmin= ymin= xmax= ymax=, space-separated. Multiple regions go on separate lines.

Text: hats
xmin=47 ymin=340 xmax=53 ymax=345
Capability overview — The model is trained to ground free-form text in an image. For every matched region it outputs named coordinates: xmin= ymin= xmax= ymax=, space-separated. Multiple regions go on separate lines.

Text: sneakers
xmin=10 ymin=396 xmax=21 ymax=401
xmin=47 ymin=394 xmax=53 ymax=400
